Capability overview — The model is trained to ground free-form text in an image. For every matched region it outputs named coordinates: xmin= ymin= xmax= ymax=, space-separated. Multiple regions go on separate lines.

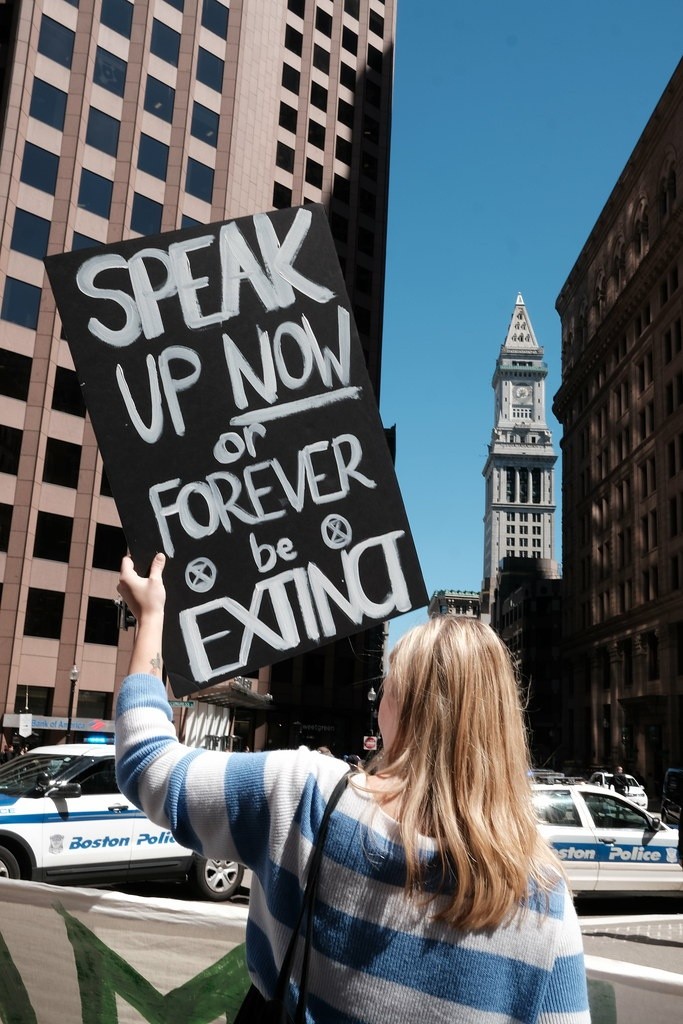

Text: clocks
xmin=513 ymin=382 xmax=533 ymax=402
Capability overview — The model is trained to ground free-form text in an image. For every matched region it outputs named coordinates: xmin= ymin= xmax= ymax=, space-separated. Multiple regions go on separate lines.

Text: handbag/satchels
xmin=233 ymin=771 xmax=361 ymax=1024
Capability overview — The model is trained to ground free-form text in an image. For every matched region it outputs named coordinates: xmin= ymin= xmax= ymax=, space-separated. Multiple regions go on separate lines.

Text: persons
xmin=635 ymin=771 xmax=644 ymax=785
xmin=608 ymin=766 xmax=629 ymax=818
xmin=298 ymin=743 xmax=361 ymax=770
xmin=115 ymin=550 xmax=590 ymax=1024
xmin=0 ymin=743 xmax=29 ymax=764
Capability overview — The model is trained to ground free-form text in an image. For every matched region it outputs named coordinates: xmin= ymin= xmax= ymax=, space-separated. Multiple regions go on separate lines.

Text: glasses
xmin=371 ymin=676 xmax=389 ymax=719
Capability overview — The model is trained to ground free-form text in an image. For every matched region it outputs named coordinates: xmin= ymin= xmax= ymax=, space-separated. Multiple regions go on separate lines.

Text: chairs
xmin=547 ymin=797 xmax=567 ymax=821
xmin=572 ymin=803 xmax=598 ymax=824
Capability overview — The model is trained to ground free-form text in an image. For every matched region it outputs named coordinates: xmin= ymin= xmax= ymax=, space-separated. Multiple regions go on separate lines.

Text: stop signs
xmin=364 ymin=735 xmax=377 ymax=750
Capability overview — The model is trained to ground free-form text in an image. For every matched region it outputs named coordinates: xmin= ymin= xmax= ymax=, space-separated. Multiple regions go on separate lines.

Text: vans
xmin=660 ymin=769 xmax=683 ymax=826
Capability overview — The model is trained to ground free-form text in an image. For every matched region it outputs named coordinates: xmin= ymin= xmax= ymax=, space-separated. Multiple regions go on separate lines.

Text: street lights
xmin=65 ymin=662 xmax=81 ymax=744
xmin=367 ymin=687 xmax=377 ymax=761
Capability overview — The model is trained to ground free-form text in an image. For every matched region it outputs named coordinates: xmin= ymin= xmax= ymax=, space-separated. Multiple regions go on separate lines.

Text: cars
xmin=590 ymin=770 xmax=648 ymax=813
xmin=530 ymin=776 xmax=683 ymax=900
xmin=0 ymin=744 xmax=245 ymax=900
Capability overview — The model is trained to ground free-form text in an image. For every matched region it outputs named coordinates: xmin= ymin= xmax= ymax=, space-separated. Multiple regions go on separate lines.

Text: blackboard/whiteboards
xmin=41 ymin=203 xmax=433 ymax=698
